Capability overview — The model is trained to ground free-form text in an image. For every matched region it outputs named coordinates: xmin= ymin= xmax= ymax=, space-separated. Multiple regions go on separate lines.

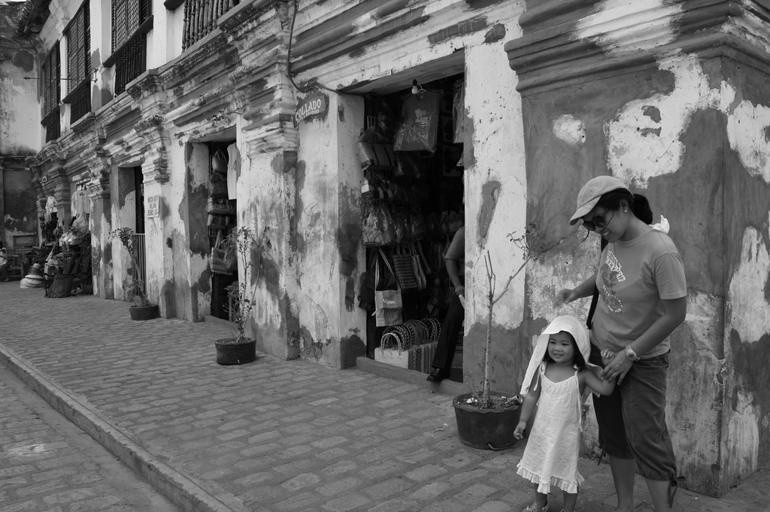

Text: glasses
xmin=580 ymin=206 xmax=615 ymax=230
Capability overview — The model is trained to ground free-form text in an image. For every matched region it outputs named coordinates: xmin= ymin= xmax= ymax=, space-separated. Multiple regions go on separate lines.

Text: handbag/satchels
xmin=204 ymin=149 xmax=234 ymax=276
xmin=357 ymin=112 xmax=442 ymax=374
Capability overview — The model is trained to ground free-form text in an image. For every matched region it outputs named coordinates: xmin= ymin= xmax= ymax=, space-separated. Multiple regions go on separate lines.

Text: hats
xmin=567 ymin=174 xmax=633 ymax=227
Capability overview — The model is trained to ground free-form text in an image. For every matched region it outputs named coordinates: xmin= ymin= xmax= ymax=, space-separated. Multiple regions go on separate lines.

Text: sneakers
xmin=522 ymin=501 xmax=550 ymax=512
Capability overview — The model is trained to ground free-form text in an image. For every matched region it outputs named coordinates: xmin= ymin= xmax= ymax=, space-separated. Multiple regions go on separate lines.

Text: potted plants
xmin=103 ymin=223 xmax=159 ymax=321
xmin=452 ymin=216 xmax=582 ymax=453
xmin=213 ymin=219 xmax=276 ymax=364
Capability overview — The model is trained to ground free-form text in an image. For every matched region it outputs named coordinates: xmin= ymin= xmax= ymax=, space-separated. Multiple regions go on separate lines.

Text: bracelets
xmin=625 ymin=344 xmax=641 ymax=362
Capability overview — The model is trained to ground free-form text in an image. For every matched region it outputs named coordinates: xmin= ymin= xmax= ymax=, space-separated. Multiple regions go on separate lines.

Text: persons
xmin=550 ymin=173 xmax=689 ymax=511
xmin=511 ymin=314 xmax=618 ymax=511
xmin=424 ymin=224 xmax=464 ymax=383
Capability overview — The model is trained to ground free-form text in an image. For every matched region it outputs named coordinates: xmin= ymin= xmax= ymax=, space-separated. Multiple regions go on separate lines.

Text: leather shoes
xmin=425 ymin=367 xmax=450 ymax=384
